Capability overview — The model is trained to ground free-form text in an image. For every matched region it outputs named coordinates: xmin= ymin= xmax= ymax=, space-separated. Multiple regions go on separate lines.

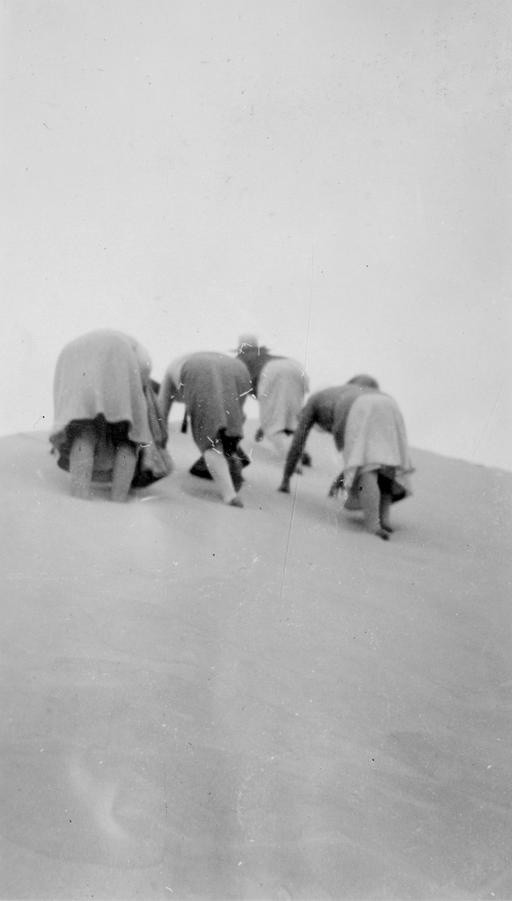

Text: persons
xmin=277 ymin=374 xmax=416 ymax=542
xmin=351 ymin=376 xmax=380 ymax=389
xmin=228 ymin=336 xmax=312 ymax=475
xmin=156 ymin=351 xmax=251 ymax=507
xmin=48 ymin=327 xmax=176 ymax=503
